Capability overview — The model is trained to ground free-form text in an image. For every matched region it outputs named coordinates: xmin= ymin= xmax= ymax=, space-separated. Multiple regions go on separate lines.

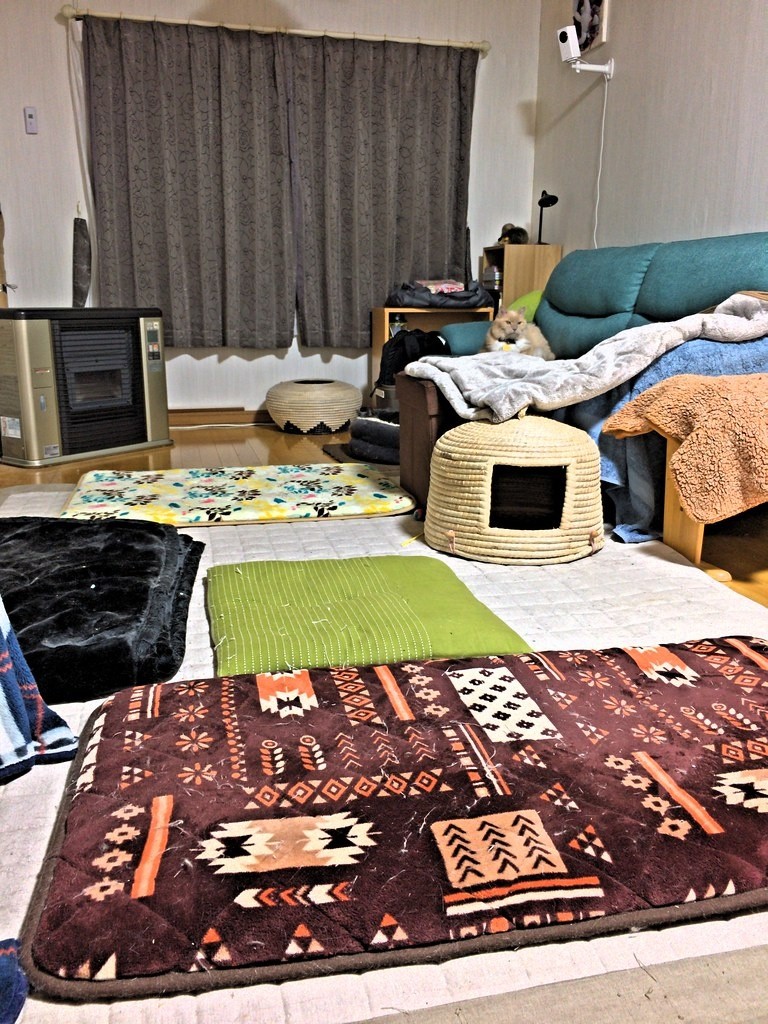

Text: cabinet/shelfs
xmin=372 ymin=306 xmax=495 ymax=410
xmin=482 ymin=245 xmax=561 ymax=309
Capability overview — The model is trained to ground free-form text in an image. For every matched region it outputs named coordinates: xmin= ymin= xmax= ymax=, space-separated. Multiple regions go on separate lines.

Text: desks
xmin=601 ymin=373 xmax=768 ymax=582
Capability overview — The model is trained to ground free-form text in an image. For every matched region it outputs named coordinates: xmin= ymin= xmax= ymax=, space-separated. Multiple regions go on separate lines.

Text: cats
xmin=477 ymin=305 xmax=556 ymax=361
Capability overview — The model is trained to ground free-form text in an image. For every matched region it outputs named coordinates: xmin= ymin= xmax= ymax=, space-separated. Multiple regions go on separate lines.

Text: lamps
xmin=535 ymin=190 xmax=559 ymax=245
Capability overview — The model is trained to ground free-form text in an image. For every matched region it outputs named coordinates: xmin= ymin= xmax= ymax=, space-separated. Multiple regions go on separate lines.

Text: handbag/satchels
xmin=372 ymin=329 xmax=451 ymax=386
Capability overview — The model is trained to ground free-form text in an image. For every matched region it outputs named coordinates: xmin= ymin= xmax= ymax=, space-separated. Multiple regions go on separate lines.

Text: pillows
xmin=507 ymin=290 xmax=542 ymax=322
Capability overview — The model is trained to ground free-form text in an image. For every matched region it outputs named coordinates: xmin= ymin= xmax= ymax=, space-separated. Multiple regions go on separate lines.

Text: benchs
xmin=393 ymin=370 xmax=458 ymax=514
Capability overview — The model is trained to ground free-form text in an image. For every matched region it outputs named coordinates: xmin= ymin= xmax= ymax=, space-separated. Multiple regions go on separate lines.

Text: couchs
xmin=439 ymin=231 xmax=768 ymax=545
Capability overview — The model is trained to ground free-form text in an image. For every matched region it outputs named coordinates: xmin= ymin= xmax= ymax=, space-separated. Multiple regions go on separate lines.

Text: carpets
xmin=0 ymin=460 xmax=768 ymax=1024
